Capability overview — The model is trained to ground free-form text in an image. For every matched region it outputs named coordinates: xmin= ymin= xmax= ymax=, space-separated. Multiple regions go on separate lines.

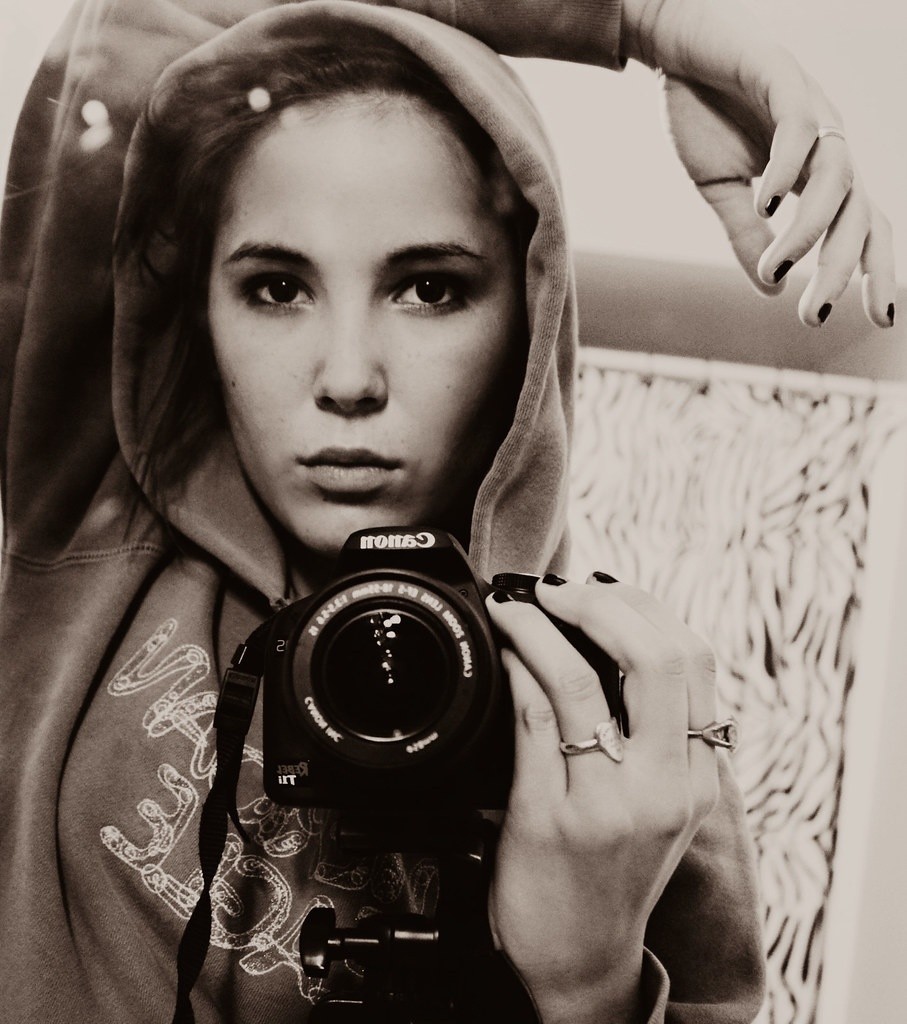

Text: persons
xmin=0 ymin=0 xmax=894 ymax=1024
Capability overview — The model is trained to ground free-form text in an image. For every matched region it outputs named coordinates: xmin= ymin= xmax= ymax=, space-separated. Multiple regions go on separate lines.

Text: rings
xmin=560 ymin=718 xmax=623 ymax=764
xmin=688 ymin=717 xmax=738 ymax=752
xmin=818 ymin=128 xmax=844 ymax=138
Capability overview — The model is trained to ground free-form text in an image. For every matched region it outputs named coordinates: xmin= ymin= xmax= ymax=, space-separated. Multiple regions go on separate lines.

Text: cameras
xmin=258 ymin=524 xmax=629 ymax=809
